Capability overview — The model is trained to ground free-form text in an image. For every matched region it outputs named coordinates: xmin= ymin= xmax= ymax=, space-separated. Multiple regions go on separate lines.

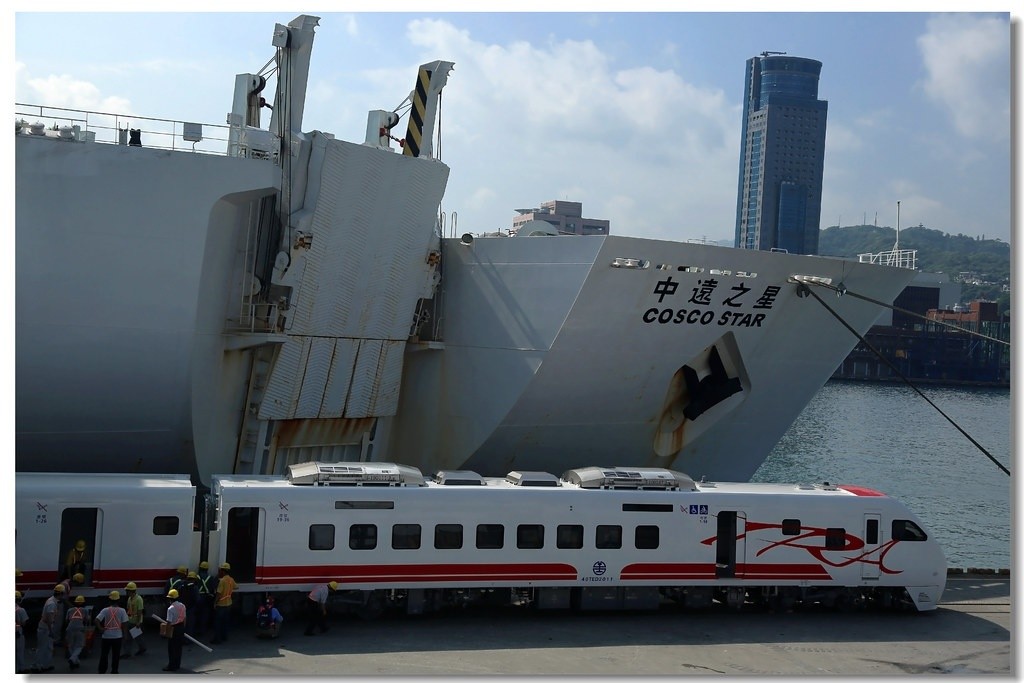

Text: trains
xmin=14 ymin=460 xmax=947 ymax=625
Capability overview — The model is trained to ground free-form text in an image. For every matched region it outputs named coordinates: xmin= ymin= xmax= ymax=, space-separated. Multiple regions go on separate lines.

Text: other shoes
xmin=19 ymin=647 xmax=180 ymax=673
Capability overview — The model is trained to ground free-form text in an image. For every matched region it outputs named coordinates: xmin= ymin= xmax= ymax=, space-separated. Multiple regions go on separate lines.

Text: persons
xmin=15 ymin=568 xmax=31 ymax=674
xmin=66 ymin=540 xmax=89 ymax=585
xmin=31 ymin=573 xmax=83 ymax=671
xmin=162 ymin=589 xmax=186 ymax=671
xmin=119 ymin=582 xmax=147 ymax=659
xmin=164 ymin=561 xmax=236 ymax=644
xmin=65 ymin=596 xmax=90 ymax=670
xmin=255 ymin=596 xmax=283 ymax=639
xmin=303 ymin=580 xmax=337 ymax=635
xmin=95 ymin=591 xmax=129 ymax=673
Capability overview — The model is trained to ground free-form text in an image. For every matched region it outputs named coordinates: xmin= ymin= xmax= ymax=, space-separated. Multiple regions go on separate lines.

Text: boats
xmin=15 ymin=0 xmax=924 ymax=495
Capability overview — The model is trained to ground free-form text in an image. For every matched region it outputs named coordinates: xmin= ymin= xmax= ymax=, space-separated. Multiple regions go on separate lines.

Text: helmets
xmin=166 ymin=589 xmax=179 ymax=599
xmin=74 ymin=595 xmax=84 ymax=603
xmin=16 ymin=591 xmax=21 ymax=599
xmin=200 ymin=562 xmax=209 ymax=569
xmin=124 ymin=583 xmax=136 ymax=591
xmin=73 ymin=573 xmax=84 ymax=583
xmin=109 ymin=591 xmax=120 ymax=600
xmin=187 ymin=572 xmax=197 ymax=578
xmin=221 ymin=563 xmax=230 ymax=570
xmin=329 ymin=581 xmax=337 ymax=591
xmin=75 ymin=541 xmax=86 ymax=551
xmin=53 ymin=584 xmax=65 ymax=593
xmin=16 ymin=569 xmax=23 ymax=576
xmin=176 ymin=566 xmax=187 ymax=575
